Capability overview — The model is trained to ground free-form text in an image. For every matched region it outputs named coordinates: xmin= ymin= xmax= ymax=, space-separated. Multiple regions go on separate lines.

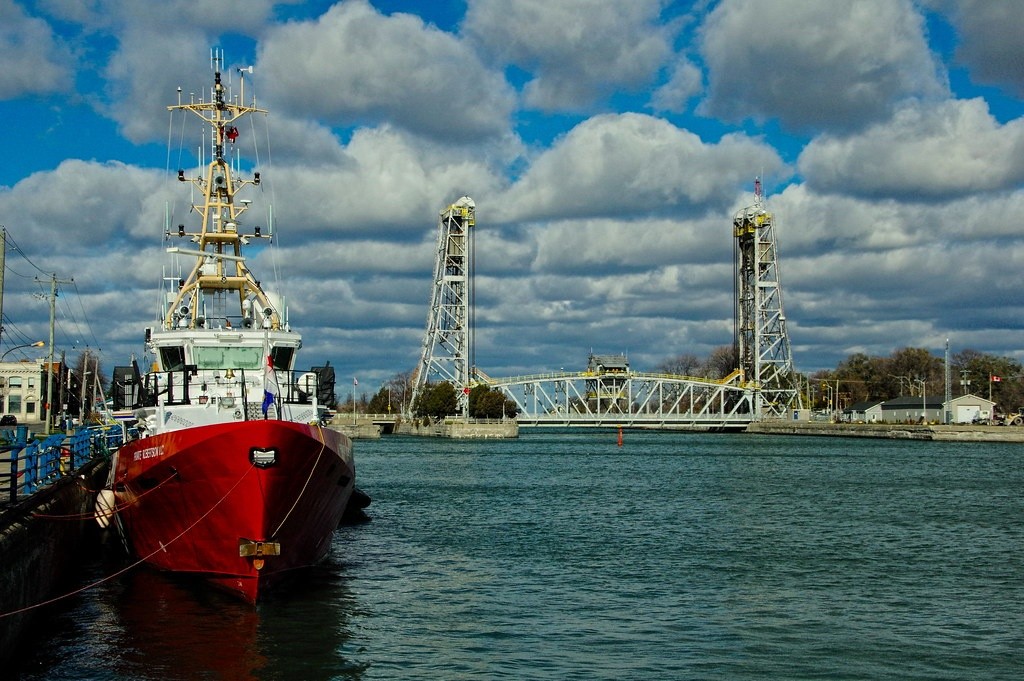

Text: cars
xmin=1 ymin=414 xmax=17 ymax=425
xmin=55 ymin=415 xmax=79 ymax=426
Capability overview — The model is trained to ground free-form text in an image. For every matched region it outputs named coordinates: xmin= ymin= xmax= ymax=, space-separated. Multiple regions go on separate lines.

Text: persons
xmin=60 ymin=416 xmax=74 ymax=432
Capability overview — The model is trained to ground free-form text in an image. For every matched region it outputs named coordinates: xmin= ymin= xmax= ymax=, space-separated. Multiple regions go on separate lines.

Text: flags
xmin=262 ymin=345 xmax=278 ymax=414
xmin=992 ymin=375 xmax=1002 ymax=382
xmin=354 ymin=377 xmax=357 ymax=385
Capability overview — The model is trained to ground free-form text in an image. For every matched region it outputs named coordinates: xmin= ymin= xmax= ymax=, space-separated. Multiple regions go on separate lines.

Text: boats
xmin=102 ymin=46 xmax=358 ymax=611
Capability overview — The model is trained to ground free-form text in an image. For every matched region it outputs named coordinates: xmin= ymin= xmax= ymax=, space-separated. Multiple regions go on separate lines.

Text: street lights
xmin=82 ymin=371 xmax=91 ymax=426
xmin=1 ymin=340 xmax=46 ymax=361
xmin=915 ymin=379 xmax=927 ymax=424
xmin=823 ymin=384 xmax=833 ymax=423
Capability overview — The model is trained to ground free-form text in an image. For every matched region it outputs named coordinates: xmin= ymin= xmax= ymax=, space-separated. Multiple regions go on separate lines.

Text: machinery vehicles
xmin=1001 ymin=407 xmax=1024 ymax=427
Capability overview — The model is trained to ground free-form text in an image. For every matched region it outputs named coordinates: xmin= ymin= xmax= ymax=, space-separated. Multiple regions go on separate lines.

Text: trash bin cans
xmin=16 ymin=426 xmax=28 ymax=445
